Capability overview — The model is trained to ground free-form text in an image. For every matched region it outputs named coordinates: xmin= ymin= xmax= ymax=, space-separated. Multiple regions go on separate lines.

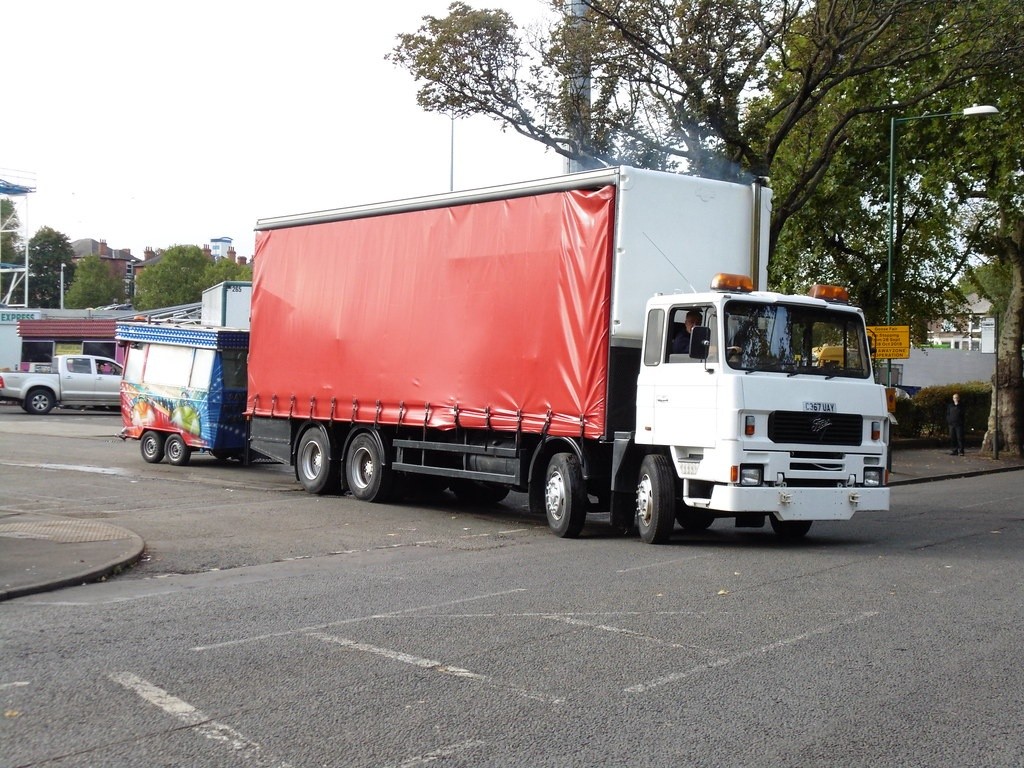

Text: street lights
xmin=60 ymin=263 xmax=67 ymax=309
xmin=887 ymin=104 xmax=1000 ymax=474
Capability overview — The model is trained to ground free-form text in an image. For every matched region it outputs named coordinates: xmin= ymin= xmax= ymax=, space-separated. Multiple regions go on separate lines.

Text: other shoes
xmin=951 ymin=453 xmax=958 ymax=455
xmin=959 ymin=453 xmax=964 ymax=456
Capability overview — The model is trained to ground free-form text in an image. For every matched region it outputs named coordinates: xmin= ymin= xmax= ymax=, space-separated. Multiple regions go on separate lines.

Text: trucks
xmin=247 ymin=165 xmax=897 ymax=544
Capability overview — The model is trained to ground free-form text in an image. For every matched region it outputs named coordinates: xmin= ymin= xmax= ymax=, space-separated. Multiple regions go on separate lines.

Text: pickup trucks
xmin=0 ymin=355 xmax=126 ymax=415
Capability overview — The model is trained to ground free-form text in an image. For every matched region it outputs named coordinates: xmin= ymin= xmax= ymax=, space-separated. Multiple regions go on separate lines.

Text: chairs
xmin=74 ymin=360 xmax=102 ymax=374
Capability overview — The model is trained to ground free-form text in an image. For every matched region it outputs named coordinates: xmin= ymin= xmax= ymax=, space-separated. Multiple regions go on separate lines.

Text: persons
xmin=97 ymin=363 xmax=105 ymax=374
xmin=672 ymin=311 xmax=703 ymax=354
xmin=947 ymin=393 xmax=967 ymax=456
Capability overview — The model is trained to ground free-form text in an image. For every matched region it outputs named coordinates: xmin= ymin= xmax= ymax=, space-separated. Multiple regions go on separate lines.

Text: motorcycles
xmin=115 ymin=316 xmax=249 ymax=465
